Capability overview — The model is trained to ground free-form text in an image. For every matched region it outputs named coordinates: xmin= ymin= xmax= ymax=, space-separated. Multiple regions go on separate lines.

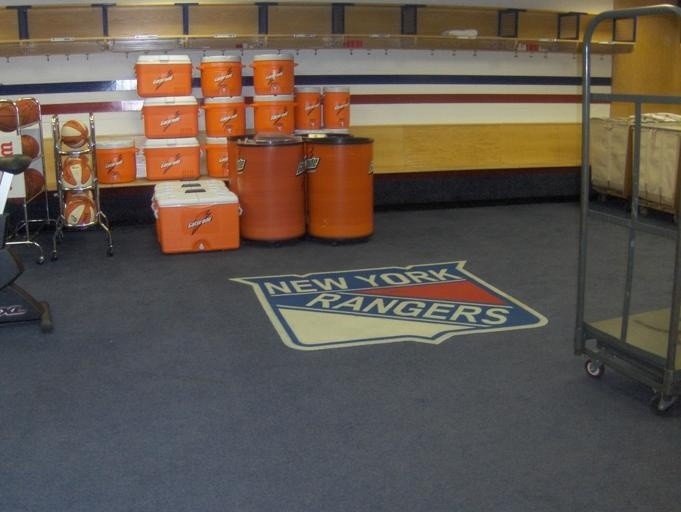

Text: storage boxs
xmin=143 ymin=136 xmax=202 ymax=181
xmin=150 ymin=191 xmax=243 ymax=255
xmin=133 ymin=54 xmax=193 ymax=98
xmin=150 ymin=179 xmax=230 ymax=243
xmin=140 ymin=96 xmax=200 ymax=139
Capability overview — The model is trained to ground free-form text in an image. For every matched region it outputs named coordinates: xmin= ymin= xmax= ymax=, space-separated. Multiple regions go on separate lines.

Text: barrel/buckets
xmin=228 ymin=132 xmax=306 ymax=244
xmin=302 ymin=131 xmax=375 ymax=242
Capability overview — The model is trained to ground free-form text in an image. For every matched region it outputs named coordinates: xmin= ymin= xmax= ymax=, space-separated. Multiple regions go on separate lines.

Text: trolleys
xmin=0 ymin=96 xmax=64 ymax=265
xmin=51 ymin=109 xmax=115 ymax=261
xmin=572 ymin=3 xmax=681 ymax=412
